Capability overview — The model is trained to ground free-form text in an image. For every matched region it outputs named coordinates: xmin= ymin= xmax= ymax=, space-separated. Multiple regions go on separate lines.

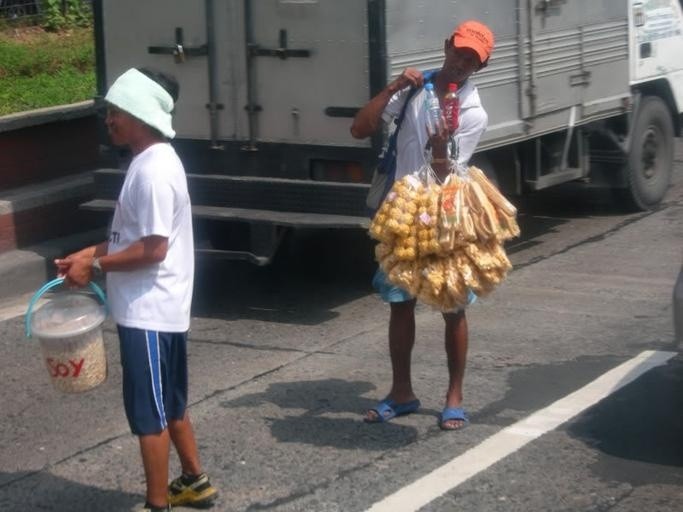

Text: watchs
xmin=91 ymin=258 xmax=103 ymax=279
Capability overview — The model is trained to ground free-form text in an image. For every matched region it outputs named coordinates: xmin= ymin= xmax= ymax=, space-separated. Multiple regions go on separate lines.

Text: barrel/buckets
xmin=24 ymin=277 xmax=110 ymax=395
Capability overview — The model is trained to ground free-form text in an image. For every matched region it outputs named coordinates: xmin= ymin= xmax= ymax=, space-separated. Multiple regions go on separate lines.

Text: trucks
xmin=77 ymin=0 xmax=683 ymax=283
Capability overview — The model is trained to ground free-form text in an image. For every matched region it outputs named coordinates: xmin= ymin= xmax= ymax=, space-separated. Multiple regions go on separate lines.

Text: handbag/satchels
xmin=367 ymin=136 xmax=396 ymax=212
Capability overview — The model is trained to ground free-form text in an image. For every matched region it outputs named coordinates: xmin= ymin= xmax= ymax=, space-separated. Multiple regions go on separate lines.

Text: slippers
xmin=364 ymin=398 xmax=420 ymax=423
xmin=440 ymin=407 xmax=468 ymax=430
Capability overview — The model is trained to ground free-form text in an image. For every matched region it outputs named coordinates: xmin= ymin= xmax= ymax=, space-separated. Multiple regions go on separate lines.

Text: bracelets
xmin=432 ymin=150 xmax=450 ymax=164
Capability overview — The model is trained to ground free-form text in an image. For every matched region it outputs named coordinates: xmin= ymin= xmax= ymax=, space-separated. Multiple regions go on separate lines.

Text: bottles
xmin=423 ymin=82 xmax=442 ymax=135
xmin=442 ymin=83 xmax=460 ymax=129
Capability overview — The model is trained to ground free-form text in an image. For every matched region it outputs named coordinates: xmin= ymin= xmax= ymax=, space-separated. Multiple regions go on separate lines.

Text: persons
xmin=53 ymin=63 xmax=222 ymax=512
xmin=347 ymin=18 xmax=497 ymax=433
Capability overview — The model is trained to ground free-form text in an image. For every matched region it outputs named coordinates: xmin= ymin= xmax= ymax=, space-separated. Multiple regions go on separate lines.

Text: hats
xmin=454 ymin=21 xmax=494 ymax=64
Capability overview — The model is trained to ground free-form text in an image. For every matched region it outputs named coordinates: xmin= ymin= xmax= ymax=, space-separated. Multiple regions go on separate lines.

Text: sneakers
xmin=166 ymin=473 xmax=219 ymax=507
